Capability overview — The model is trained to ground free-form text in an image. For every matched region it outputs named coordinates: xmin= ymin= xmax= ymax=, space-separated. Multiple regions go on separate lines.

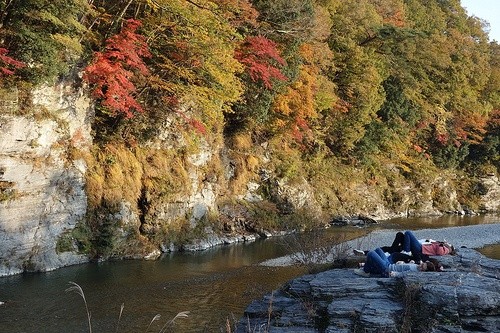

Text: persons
xmin=351 ymin=230 xmax=459 ymax=278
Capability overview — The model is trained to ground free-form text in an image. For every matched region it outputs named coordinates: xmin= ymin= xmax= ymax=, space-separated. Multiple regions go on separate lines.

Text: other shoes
xmin=354 ymin=268 xmax=371 ymax=277
xmin=401 ymin=250 xmax=412 ymax=255
xmin=353 ymin=248 xmax=365 ymax=255
